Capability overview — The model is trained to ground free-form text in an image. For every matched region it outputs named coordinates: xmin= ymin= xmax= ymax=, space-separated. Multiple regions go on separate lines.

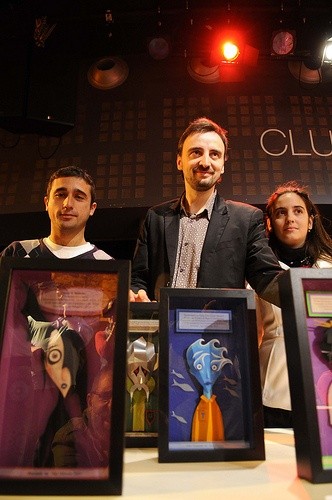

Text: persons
xmin=125 ymin=117 xmax=287 ymax=307
xmin=247 ymin=186 xmax=332 ymax=428
xmin=1 ymin=166 xmax=111 ymax=260
